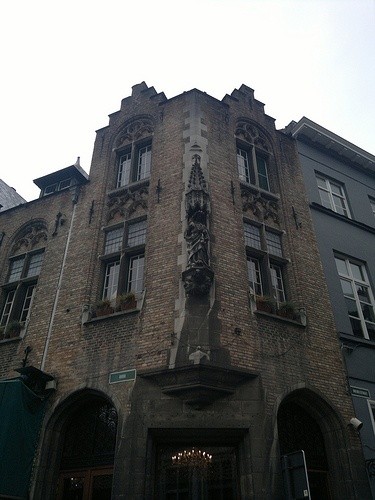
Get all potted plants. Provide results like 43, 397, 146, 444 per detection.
256, 295, 276, 313
116, 292, 138, 312
92, 299, 113, 318
5, 321, 25, 338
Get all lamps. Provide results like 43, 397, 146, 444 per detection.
347, 417, 364, 431
